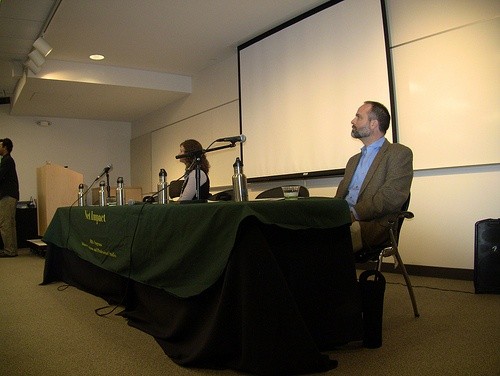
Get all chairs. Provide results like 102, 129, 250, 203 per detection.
360, 192, 421, 319
256, 184, 310, 199
211, 189, 234, 200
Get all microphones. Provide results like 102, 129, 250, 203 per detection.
97, 163, 112, 179
217, 134, 246, 142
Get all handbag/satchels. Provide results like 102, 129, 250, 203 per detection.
357, 270, 386, 348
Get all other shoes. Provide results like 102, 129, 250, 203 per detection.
0, 251, 18, 257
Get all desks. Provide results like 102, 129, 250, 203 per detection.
91, 186, 143, 204
39, 195, 362, 376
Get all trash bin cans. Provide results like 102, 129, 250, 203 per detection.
0, 200, 38, 250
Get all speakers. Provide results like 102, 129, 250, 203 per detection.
474, 218, 500, 294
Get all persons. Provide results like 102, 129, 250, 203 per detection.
0, 138, 19, 257
178, 139, 210, 200
334, 101, 414, 251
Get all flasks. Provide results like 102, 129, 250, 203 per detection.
78, 183, 87, 206
98, 181, 107, 207
157, 169, 169, 204
232, 157, 248, 202
116, 177, 126, 206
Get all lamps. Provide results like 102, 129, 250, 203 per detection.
33, 32, 53, 57
27, 49, 46, 66
23, 59, 43, 74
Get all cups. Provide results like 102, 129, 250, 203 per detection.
281, 185, 300, 201
108, 196, 116, 207
